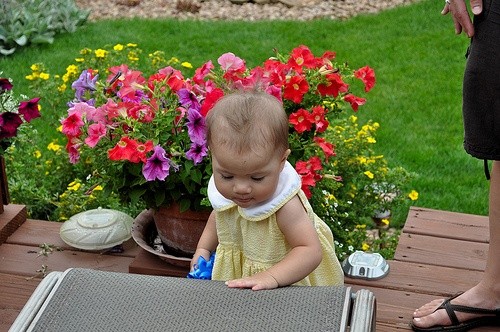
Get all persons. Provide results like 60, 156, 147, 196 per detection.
174, 90, 348, 290
402, 0, 500, 332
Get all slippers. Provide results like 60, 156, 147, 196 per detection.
409, 292, 500, 332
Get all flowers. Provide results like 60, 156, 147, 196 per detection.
3, 36, 410, 264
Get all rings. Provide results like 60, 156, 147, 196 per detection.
446, 0, 451, 6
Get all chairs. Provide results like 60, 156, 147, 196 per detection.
7, 266, 382, 332
